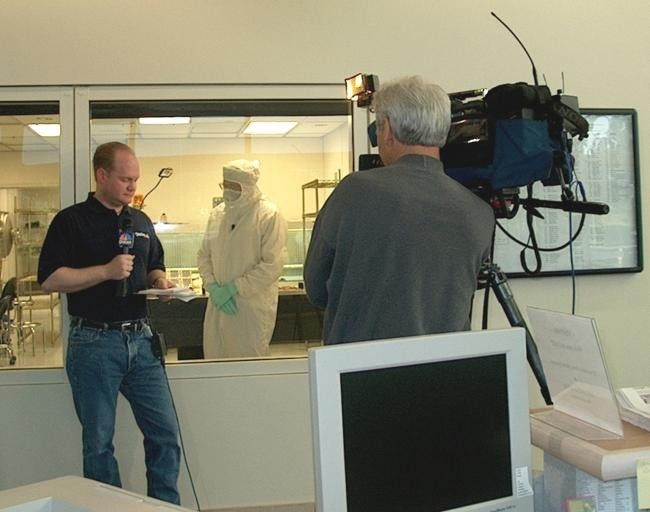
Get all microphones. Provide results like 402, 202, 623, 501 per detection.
116, 219, 134, 297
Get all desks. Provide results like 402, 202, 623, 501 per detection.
531, 405, 650, 512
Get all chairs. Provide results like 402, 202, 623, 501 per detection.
0, 277, 45, 365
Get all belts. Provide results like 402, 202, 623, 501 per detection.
72, 318, 148, 331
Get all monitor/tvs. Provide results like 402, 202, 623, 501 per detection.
307, 327, 534, 512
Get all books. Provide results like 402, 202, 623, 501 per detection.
530, 388, 650, 511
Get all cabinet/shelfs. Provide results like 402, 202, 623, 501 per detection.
302, 170, 342, 261
14, 196, 63, 347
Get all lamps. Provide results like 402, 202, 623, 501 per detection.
344, 74, 380, 107
139, 167, 173, 211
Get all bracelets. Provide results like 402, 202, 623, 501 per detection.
152, 274, 167, 289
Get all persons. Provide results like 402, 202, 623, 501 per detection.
192, 154, 289, 360
293, 75, 496, 346
33, 141, 184, 505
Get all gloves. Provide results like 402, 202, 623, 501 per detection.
206, 283, 238, 315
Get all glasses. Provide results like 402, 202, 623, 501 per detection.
219, 182, 239, 190
